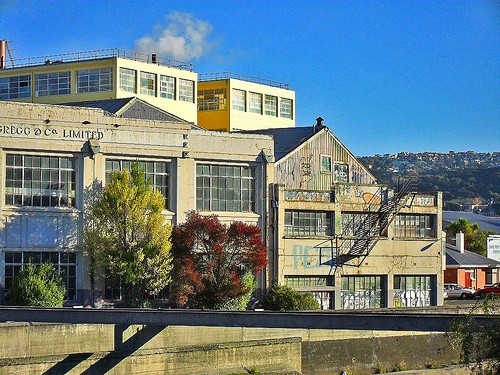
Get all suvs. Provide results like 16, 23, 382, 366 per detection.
480, 282, 500, 298
444, 284, 476, 300
